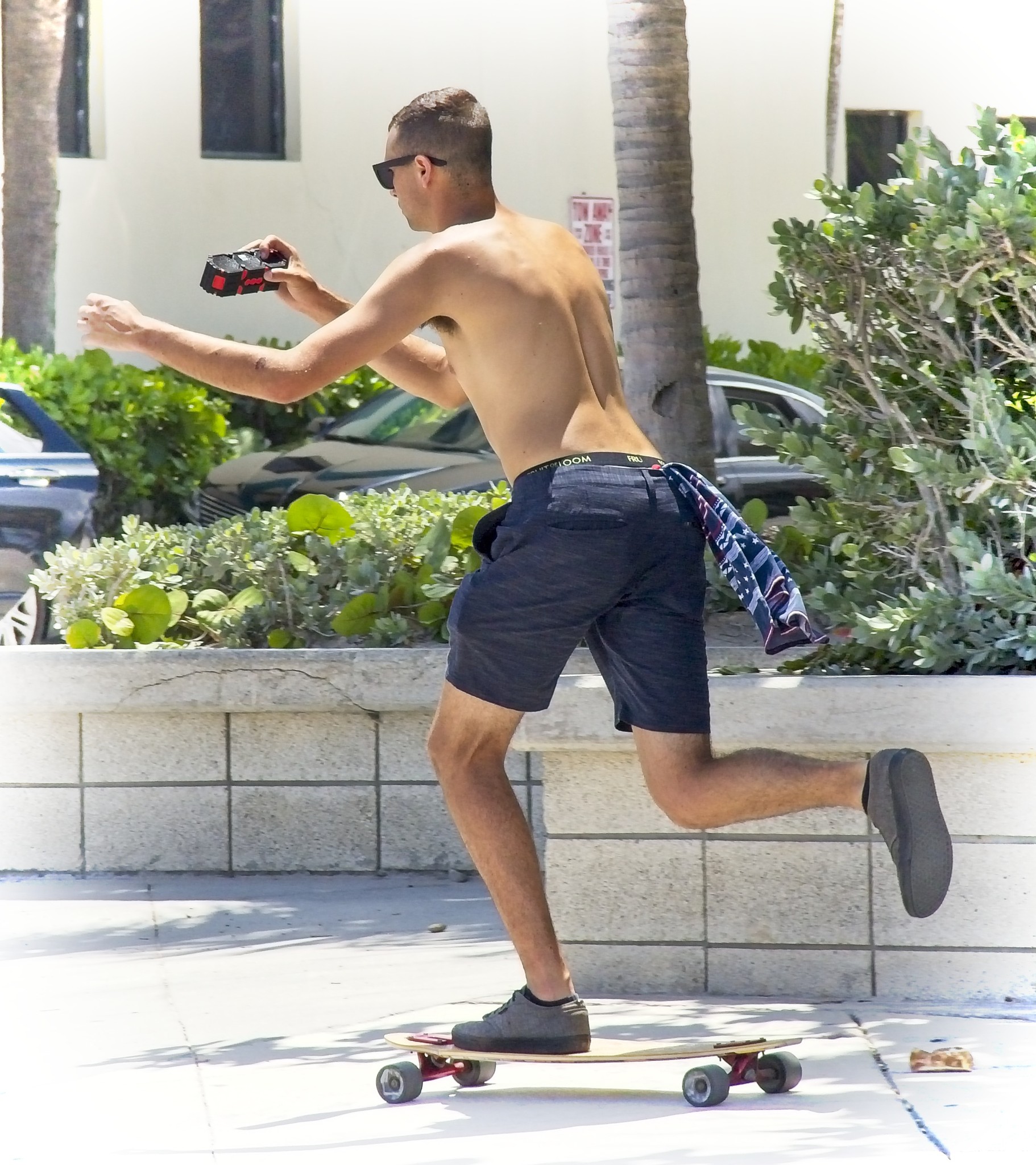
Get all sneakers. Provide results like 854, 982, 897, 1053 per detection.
864, 749, 953, 919
451, 985, 591, 1053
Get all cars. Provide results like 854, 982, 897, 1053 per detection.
192, 353, 828, 530
0, 381, 106, 648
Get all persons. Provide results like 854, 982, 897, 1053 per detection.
74, 89, 953, 1054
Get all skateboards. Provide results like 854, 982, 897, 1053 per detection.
376, 1032, 803, 1107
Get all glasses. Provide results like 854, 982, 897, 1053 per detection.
373, 155, 447, 190
